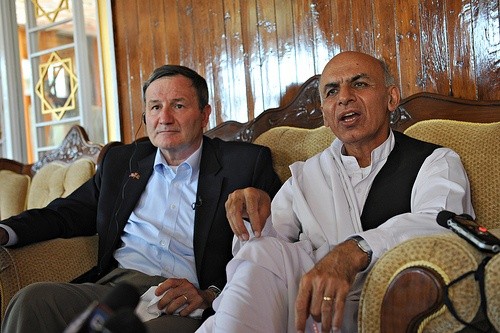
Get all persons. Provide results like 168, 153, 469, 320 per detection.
196, 50, 476, 333
0, 64, 281, 333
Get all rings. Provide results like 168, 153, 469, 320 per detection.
183, 294, 188, 303
323, 296, 331, 301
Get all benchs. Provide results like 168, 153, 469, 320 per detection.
0, 74, 500, 333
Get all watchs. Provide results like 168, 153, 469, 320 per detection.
349, 235, 372, 270
209, 288, 221, 298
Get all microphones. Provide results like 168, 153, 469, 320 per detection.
436, 210, 500, 252
78, 282, 147, 332
